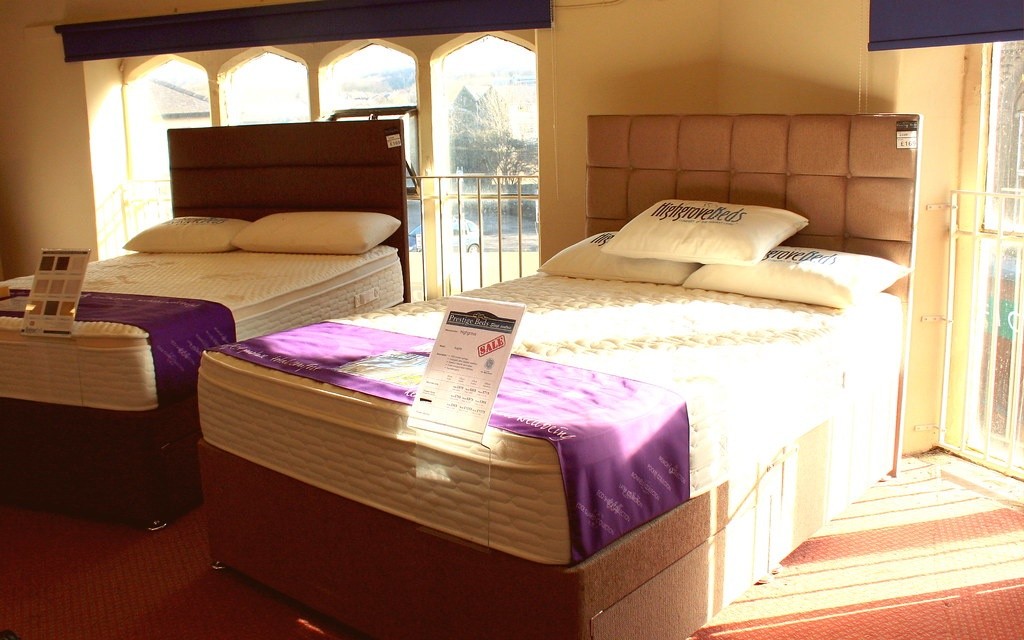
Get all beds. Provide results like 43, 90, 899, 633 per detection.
0, 121, 411, 530
197, 111, 922, 640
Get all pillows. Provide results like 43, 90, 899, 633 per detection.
123, 217, 252, 252
601, 199, 809, 266
232, 211, 401, 254
683, 246, 914, 314
537, 231, 701, 286
